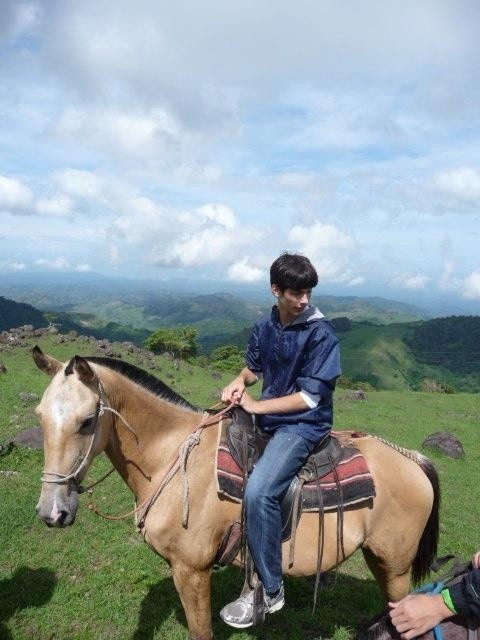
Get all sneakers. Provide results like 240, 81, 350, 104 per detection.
219, 581, 286, 629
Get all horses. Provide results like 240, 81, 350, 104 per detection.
33, 345, 442, 640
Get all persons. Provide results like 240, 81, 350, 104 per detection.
213, 251, 344, 629
383, 550, 480, 640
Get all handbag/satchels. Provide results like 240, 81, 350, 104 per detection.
354, 554, 480, 640
